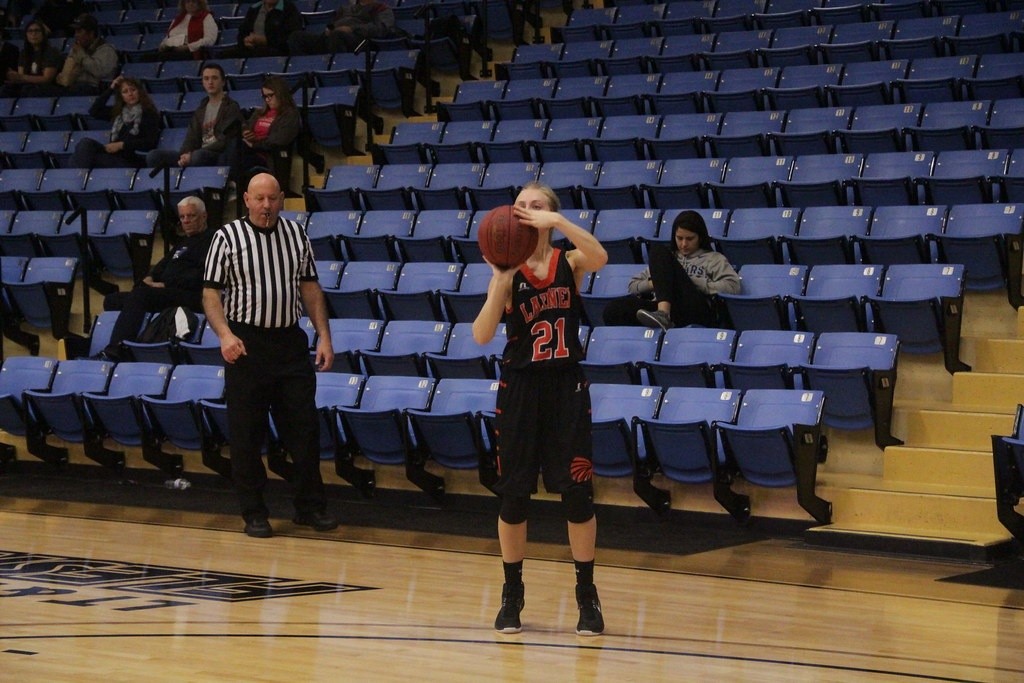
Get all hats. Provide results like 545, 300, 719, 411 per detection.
68, 13, 98, 29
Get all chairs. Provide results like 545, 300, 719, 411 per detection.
0, 0, 1024, 537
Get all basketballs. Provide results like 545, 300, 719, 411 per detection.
478, 205, 539, 266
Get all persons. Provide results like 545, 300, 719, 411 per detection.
628, 210, 741, 332
146, 63, 240, 168
103, 195, 219, 313
214, 77, 300, 202
0, 0, 119, 96
288, 0, 395, 57
201, 172, 338, 538
158, 0, 219, 61
75, 76, 166, 168
471, 181, 608, 636
237, 0, 305, 58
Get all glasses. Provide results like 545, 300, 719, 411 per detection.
262, 93, 275, 99
26, 29, 41, 34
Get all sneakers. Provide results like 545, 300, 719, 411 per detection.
293, 509, 339, 532
637, 309, 668, 333
495, 581, 525, 633
245, 519, 274, 539
574, 583, 604, 635
